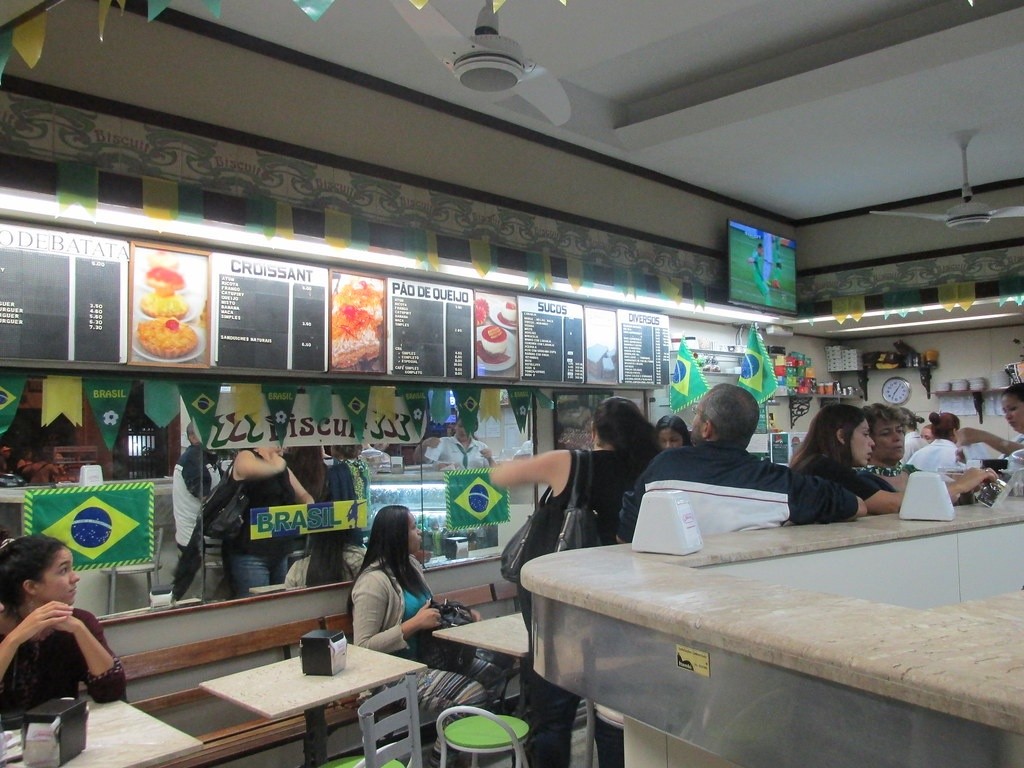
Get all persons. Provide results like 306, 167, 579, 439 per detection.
959, 383, 1024, 466
327, 445, 370, 583
414, 415, 457, 463
906, 410, 960, 504
616, 383, 867, 543
172, 420, 231, 600
921, 424, 935, 445
346, 504, 509, 768
789, 404, 999, 515
221, 447, 314, 598
489, 396, 667, 768
432, 414, 497, 470
899, 407, 928, 464
0, 530, 127, 713
853, 404, 974, 506
655, 414, 691, 450
363, 443, 390, 462
748, 231, 783, 307
18, 436, 60, 484
283, 446, 329, 586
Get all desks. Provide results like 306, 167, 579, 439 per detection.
432, 613, 530, 658
96, 597, 202, 622
248, 583, 285, 596
0, 689, 203, 768
199, 645, 428, 768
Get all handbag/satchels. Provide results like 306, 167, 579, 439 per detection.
425, 600, 477, 674
196, 449, 264, 542
501, 448, 601, 584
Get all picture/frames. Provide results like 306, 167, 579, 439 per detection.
553, 391, 614, 450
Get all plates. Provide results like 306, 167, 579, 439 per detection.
132, 258, 209, 363
476, 298, 519, 372
936, 370, 1011, 391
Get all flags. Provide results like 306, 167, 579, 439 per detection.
670, 337, 712, 414
445, 470, 510, 531
737, 326, 778, 404
23, 482, 154, 570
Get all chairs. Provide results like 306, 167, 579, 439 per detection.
357, 672, 424, 768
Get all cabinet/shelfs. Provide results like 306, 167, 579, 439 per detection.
669, 348, 745, 377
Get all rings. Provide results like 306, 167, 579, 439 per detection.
985, 468, 988, 471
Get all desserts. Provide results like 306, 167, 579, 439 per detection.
481, 301, 519, 355
138, 267, 198, 359
331, 279, 383, 367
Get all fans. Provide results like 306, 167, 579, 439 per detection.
390, 0, 572, 127
868, 132, 1024, 230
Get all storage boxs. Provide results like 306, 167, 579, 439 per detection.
775, 351, 816, 396
824, 345, 863, 372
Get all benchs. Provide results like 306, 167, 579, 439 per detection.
77, 580, 522, 768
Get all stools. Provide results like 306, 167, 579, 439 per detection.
198, 531, 225, 604
101, 527, 164, 615
437, 705, 530, 768
318, 741, 413, 768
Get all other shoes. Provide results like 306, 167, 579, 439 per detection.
430, 748, 460, 768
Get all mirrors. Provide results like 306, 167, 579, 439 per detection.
0, 374, 540, 627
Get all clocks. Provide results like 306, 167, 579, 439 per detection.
881, 376, 912, 406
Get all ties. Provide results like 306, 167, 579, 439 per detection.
457, 444, 473, 468
